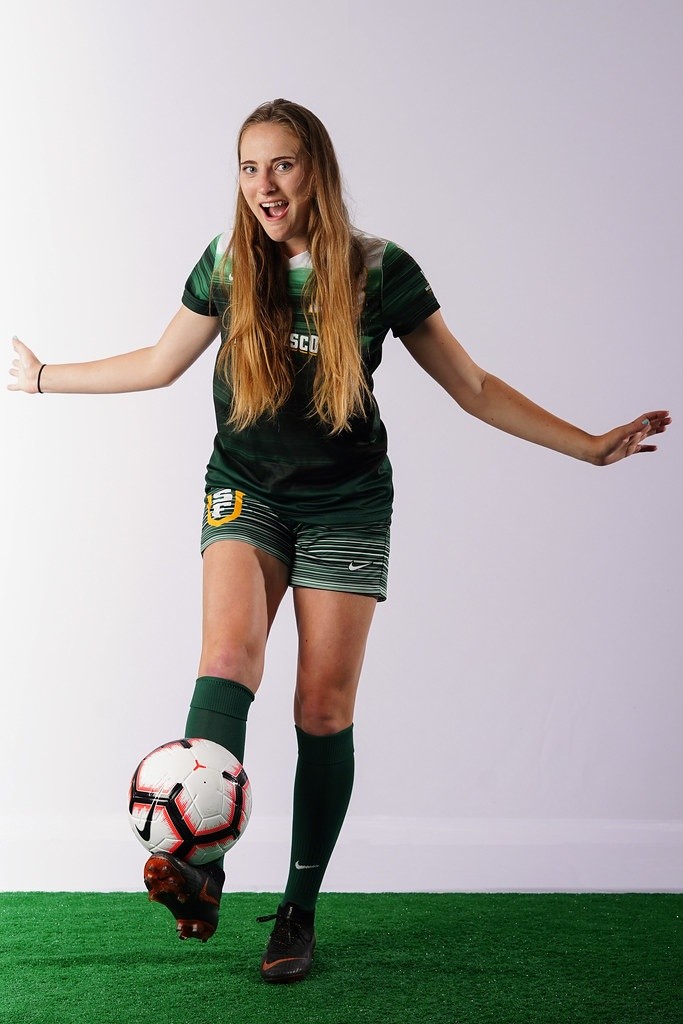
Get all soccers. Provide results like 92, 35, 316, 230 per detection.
129, 739, 253, 865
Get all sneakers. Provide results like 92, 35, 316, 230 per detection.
142, 851, 227, 940
258, 905, 318, 980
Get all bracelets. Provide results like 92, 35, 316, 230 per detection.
37, 364, 47, 394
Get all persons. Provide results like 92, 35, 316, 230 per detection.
7, 97, 672, 985
